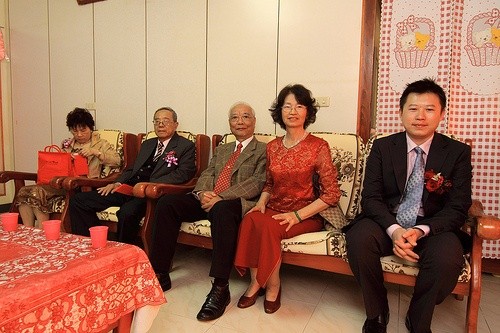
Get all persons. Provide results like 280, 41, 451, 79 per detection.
233, 86, 341, 315
147, 102, 270, 322
346, 78, 472, 333
67, 107, 196, 241
14, 108, 121, 231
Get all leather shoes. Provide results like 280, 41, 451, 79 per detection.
197, 280, 231, 320
158, 274, 171, 291
363, 305, 390, 333
405, 321, 432, 333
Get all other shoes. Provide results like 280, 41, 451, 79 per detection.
264, 278, 281, 313
237, 287, 263, 308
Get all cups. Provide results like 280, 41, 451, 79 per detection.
42, 219, 61, 240
89, 226, 109, 248
1, 213, 19, 231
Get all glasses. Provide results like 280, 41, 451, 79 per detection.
71, 128, 91, 134
230, 115, 254, 122
152, 120, 176, 126
280, 103, 308, 112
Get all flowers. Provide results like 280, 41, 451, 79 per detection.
424, 169, 452, 195
162, 149, 178, 167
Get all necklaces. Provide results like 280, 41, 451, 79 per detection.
284, 129, 308, 149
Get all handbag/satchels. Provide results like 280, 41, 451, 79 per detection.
71, 153, 89, 175
37, 145, 72, 185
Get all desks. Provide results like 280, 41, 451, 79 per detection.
0, 223, 167, 333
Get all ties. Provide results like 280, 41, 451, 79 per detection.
396, 147, 426, 229
213, 144, 243, 195
153, 141, 164, 162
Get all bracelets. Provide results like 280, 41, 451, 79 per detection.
293, 210, 302, 223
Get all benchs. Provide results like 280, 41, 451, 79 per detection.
146, 131, 500, 333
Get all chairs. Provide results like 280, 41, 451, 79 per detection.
0, 129, 211, 255
